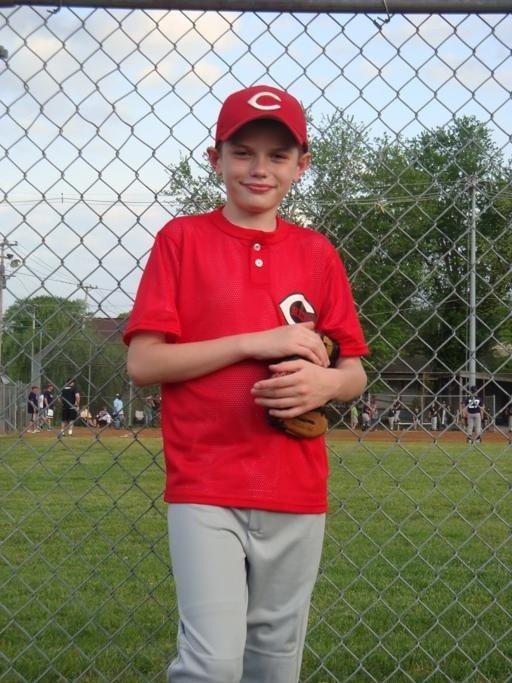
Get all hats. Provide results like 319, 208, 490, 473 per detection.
215, 85, 308, 156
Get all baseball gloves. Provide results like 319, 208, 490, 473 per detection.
267, 334, 340, 439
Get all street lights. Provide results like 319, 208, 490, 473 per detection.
0, 241, 20, 313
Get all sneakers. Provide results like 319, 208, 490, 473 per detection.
62, 434, 72, 437
27, 427, 53, 432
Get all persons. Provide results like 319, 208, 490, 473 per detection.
347, 382, 491, 444
24, 376, 160, 439
118, 85, 372, 683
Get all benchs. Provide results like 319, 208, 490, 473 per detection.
394, 422, 433, 432
